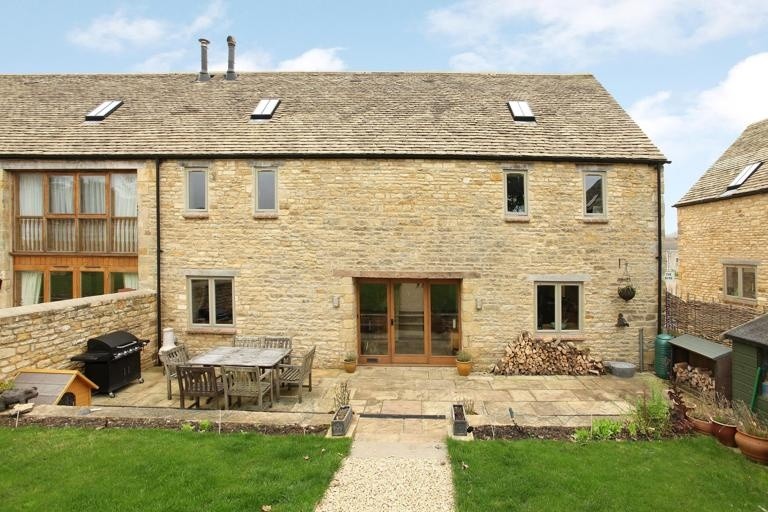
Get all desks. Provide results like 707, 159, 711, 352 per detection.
184, 346, 293, 406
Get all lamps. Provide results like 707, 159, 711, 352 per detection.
331, 295, 340, 309
474, 297, 483, 311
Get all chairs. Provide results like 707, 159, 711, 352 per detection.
260, 338, 292, 388
220, 364, 273, 412
175, 365, 223, 411
231, 335, 262, 350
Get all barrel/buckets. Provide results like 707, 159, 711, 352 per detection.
653, 333, 674, 380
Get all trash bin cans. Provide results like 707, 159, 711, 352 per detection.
655, 334, 673, 380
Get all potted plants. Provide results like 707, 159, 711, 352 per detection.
616, 258, 636, 302
330, 379, 353, 436
708, 395, 736, 447
730, 399, 767, 464
451, 404, 467, 435
684, 399, 712, 437
342, 350, 357, 374
454, 349, 472, 377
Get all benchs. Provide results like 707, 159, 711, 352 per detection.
160, 344, 193, 402
275, 345, 316, 404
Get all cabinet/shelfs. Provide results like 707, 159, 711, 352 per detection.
83, 351, 145, 399
